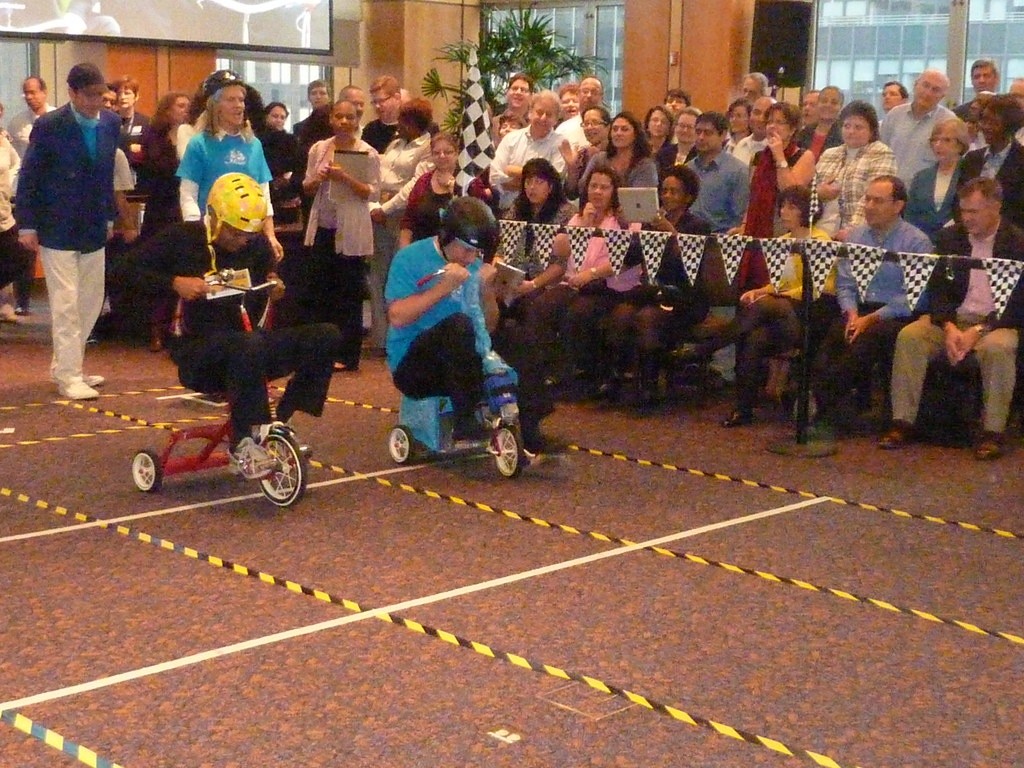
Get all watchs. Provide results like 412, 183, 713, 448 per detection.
775, 160, 789, 171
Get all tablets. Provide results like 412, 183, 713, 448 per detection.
618, 187, 659, 223
492, 261, 525, 307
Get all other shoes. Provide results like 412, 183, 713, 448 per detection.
57, 378, 99, 401
879, 421, 914, 450
518, 354, 862, 428
83, 375, 104, 387
150, 323, 162, 352
976, 429, 1005, 461
521, 428, 549, 460
451, 421, 490, 449
15, 303, 32, 315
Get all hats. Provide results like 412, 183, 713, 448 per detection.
66, 63, 109, 97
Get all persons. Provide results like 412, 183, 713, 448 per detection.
2, 55, 1024, 479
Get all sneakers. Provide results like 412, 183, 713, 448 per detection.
226, 435, 274, 479
276, 422, 313, 458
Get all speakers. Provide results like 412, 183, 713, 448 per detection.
750, 0, 816, 88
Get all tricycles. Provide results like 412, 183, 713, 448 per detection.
388, 265, 533, 478
132, 279, 312, 509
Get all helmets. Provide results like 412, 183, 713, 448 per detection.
207, 172, 266, 232
202, 69, 246, 101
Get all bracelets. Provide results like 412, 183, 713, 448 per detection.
445, 179, 455, 188
591, 267, 598, 281
531, 280, 539, 292
975, 323, 986, 336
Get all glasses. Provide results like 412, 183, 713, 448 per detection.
694, 128, 719, 136
371, 93, 393, 105
929, 135, 960, 145
580, 119, 608, 128
677, 122, 696, 131
